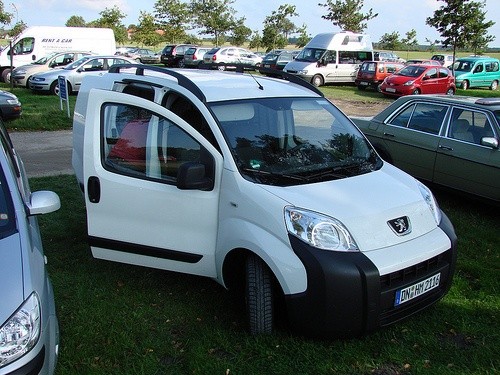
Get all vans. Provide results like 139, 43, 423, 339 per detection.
283, 31, 375, 88
0, 26, 116, 84
355, 61, 408, 90
448, 56, 500, 91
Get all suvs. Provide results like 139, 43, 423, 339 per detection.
183, 47, 217, 68
159, 44, 199, 67
202, 47, 263, 72
259, 53, 306, 78
75, 64, 458, 339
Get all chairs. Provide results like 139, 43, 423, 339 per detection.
92, 59, 102, 70
450, 118, 477, 143
62, 54, 72, 65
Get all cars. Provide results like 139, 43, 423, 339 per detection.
381, 65, 456, 98
0, 90, 64, 375
10, 51, 105, 88
28, 55, 143, 97
332, 95, 500, 202
374, 51, 407, 65
406, 59, 443, 69
124, 48, 160, 64
431, 55, 468, 69
254, 50, 302, 60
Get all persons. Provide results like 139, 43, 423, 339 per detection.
107, 106, 150, 169
